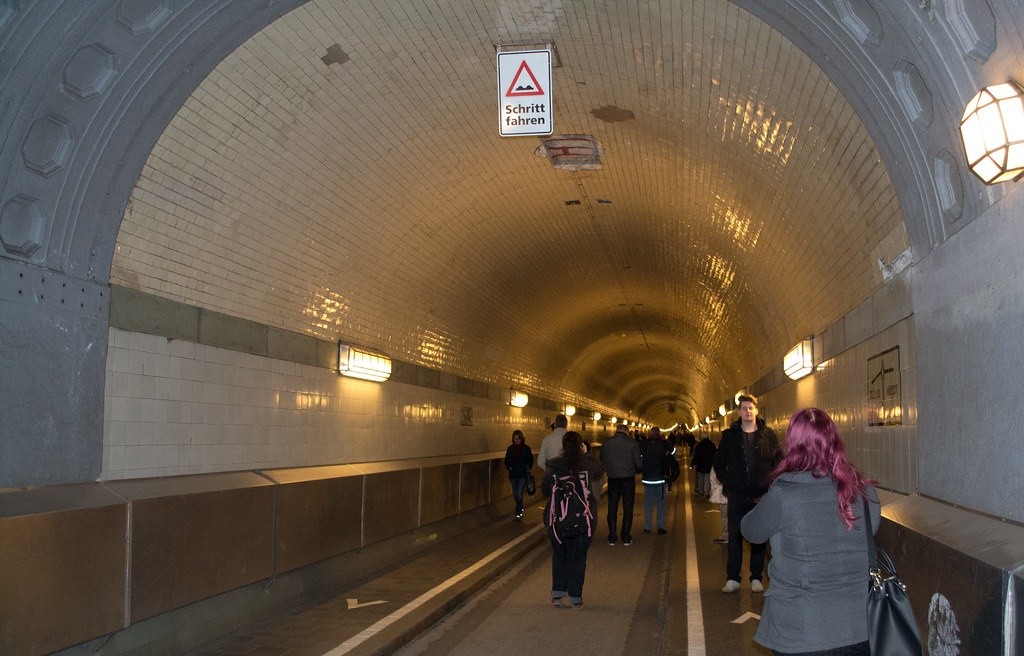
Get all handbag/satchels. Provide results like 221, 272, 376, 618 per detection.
526, 469, 536, 496
868, 568, 923, 656
662, 451, 681, 483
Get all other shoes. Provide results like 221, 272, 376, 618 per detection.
608, 541, 615, 545
624, 540, 633, 546
572, 602, 583, 609
752, 579, 764, 593
713, 538, 729, 543
552, 597, 562, 605
705, 495, 710, 501
722, 579, 740, 593
514, 512, 522, 521
644, 529, 651, 533
698, 493, 703, 497
658, 528, 666, 534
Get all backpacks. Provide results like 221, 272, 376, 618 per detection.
548, 475, 593, 544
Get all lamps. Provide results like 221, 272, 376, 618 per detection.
560, 401, 730, 432
784, 335, 814, 380
510, 390, 529, 408
735, 385, 750, 406
958, 78, 1024, 186
336, 342, 391, 382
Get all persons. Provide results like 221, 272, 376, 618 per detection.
627, 428, 647, 443
667, 427, 696, 455
540, 430, 603, 610
504, 430, 534, 523
740, 409, 880, 656
713, 400, 785, 597
690, 431, 719, 502
639, 426, 682, 536
598, 423, 643, 547
707, 466, 729, 545
536, 413, 569, 472
